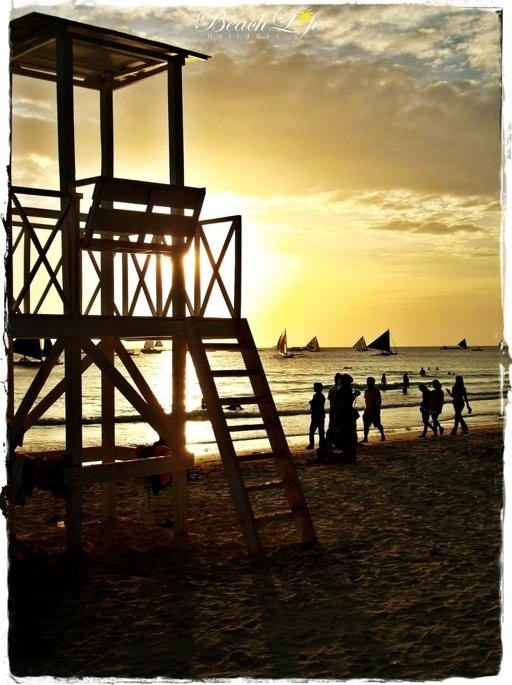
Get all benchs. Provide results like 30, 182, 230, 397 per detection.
78, 177, 205, 258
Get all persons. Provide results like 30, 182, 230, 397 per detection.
306, 366, 473, 451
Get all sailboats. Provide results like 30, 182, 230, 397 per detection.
140, 338, 166, 353
352, 328, 399, 356
13, 338, 63, 365
440, 339, 470, 352
276, 328, 320, 358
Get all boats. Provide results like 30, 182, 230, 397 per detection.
114, 349, 140, 356
